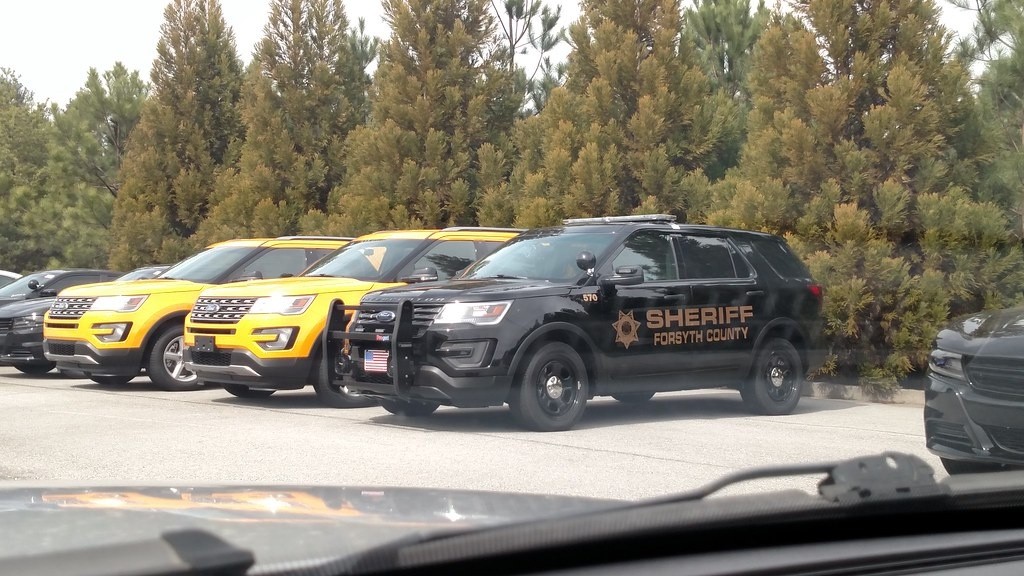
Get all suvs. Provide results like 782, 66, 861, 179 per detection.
178, 225, 532, 408
923, 303, 1024, 477
1, 267, 130, 377
328, 214, 827, 433
42, 235, 389, 393
